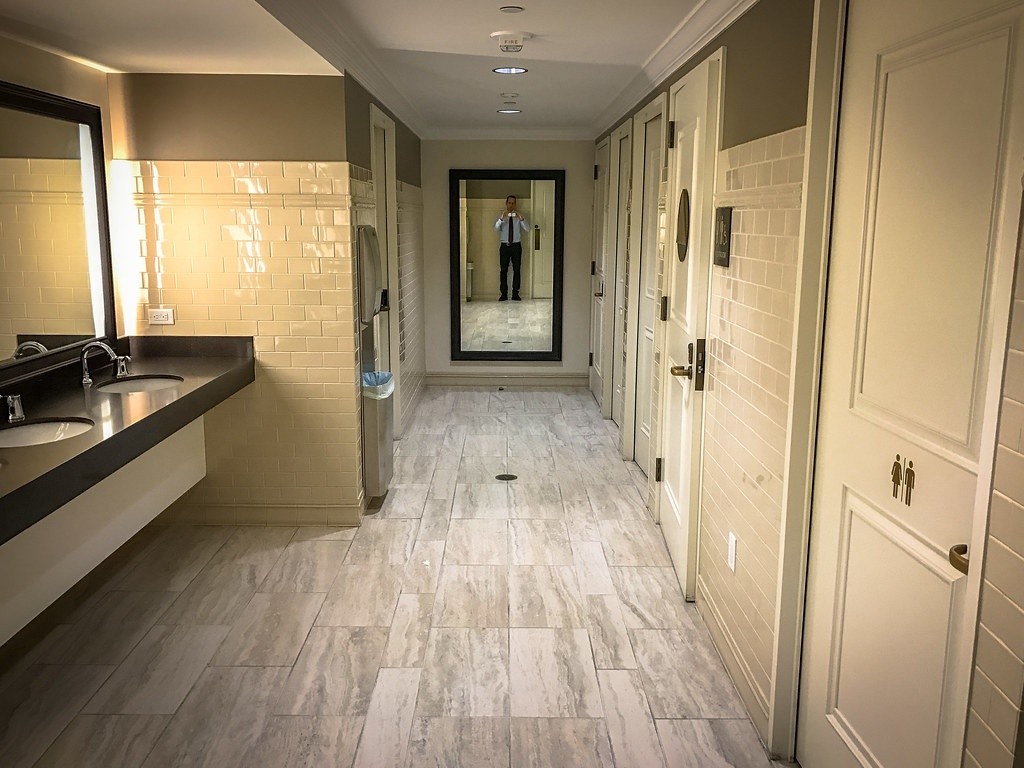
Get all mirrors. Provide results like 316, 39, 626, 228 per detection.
0, 80, 120, 388
448, 168, 566, 362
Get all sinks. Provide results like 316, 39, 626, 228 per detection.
93, 374, 184, 393
0, 416, 96, 448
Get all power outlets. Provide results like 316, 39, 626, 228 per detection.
726, 532, 738, 573
148, 308, 175, 325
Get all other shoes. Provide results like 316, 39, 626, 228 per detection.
499, 295, 509, 301
512, 295, 521, 301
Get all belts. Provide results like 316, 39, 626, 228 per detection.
501, 242, 521, 246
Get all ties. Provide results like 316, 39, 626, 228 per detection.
508, 217, 514, 243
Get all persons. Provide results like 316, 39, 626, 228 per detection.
495, 196, 530, 301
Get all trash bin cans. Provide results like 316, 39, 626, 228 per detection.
466, 263, 472, 303
363, 372, 394, 498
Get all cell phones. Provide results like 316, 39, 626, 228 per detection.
508, 213, 516, 217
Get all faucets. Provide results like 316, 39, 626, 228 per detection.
80, 341, 117, 384
14, 340, 48, 356
